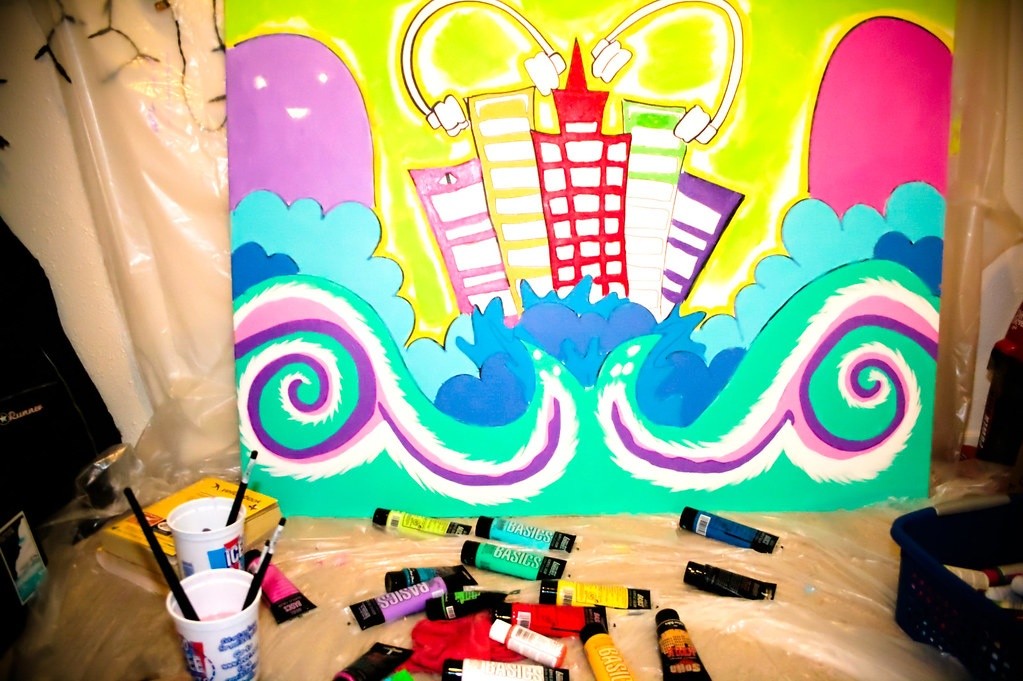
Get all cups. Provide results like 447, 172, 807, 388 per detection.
166, 496, 246, 576
165, 568, 262, 681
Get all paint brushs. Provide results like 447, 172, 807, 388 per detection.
226, 450, 259, 529
242, 518, 286, 611
253, 539, 271, 577
123, 486, 201, 622
